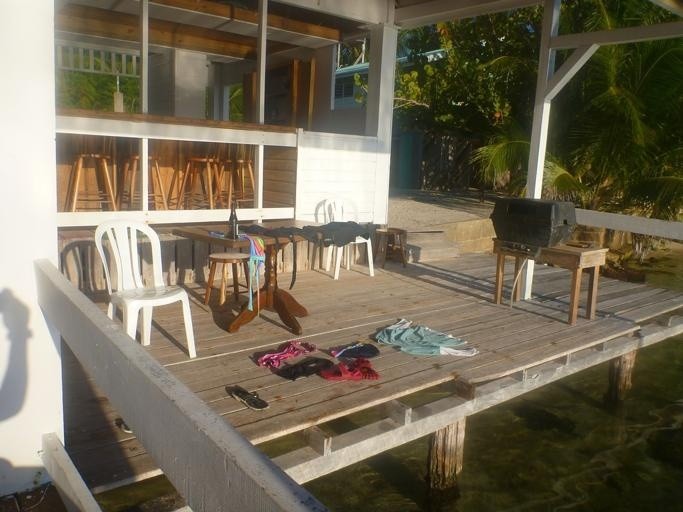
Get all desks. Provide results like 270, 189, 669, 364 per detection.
173, 221, 368, 334
490, 238, 609, 327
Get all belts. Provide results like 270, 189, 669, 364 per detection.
288, 233, 298, 290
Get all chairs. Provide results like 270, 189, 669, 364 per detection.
215, 144, 254, 208
93, 218, 198, 360
325, 197, 374, 280
176, 141, 224, 210
69, 133, 118, 212
118, 138, 169, 211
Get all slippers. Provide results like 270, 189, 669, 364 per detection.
230, 386, 270, 412
320, 364, 381, 382
283, 358, 332, 380
338, 340, 381, 357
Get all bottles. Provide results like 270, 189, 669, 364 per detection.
228, 203, 238, 239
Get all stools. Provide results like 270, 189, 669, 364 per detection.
205, 253, 250, 309
374, 227, 407, 270
